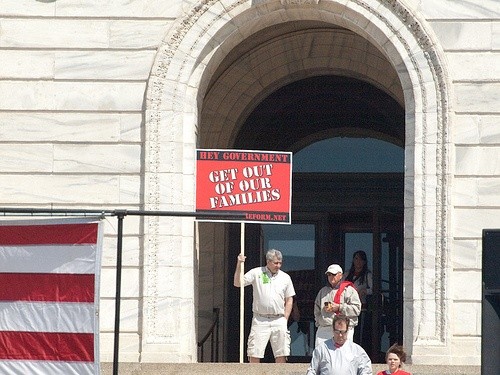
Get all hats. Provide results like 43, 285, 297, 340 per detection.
325, 264, 343, 274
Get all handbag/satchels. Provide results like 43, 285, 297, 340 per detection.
367, 295, 374, 309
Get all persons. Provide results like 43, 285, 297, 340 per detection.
342, 251, 374, 363
233, 248, 296, 363
307, 312, 374, 375
313, 264, 362, 349
376, 342, 411, 375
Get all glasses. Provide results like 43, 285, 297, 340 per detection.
334, 329, 347, 334
328, 273, 338, 276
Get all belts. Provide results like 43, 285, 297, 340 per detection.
253, 313, 284, 318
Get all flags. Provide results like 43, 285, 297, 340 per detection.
0, 216, 108, 375
